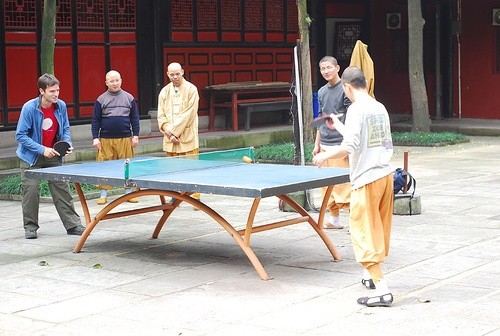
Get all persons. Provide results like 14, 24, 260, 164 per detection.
311, 56, 352, 229
15, 73, 86, 239
91, 70, 140, 204
312, 66, 393, 306
157, 62, 201, 210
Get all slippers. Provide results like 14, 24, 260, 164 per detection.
323, 220, 343, 229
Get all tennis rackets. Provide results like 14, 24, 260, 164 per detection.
53, 141, 70, 157
309, 113, 343, 128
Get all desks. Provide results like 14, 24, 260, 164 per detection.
223, 96, 292, 131
204, 80, 291, 132
25, 155, 351, 281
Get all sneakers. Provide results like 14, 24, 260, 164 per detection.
128, 196, 138, 203
97, 197, 107, 204
67, 225, 86, 235
25, 229, 37, 239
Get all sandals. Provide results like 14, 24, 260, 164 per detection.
357, 293, 393, 307
362, 279, 376, 289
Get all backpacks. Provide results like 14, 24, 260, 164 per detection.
394, 168, 415, 199
